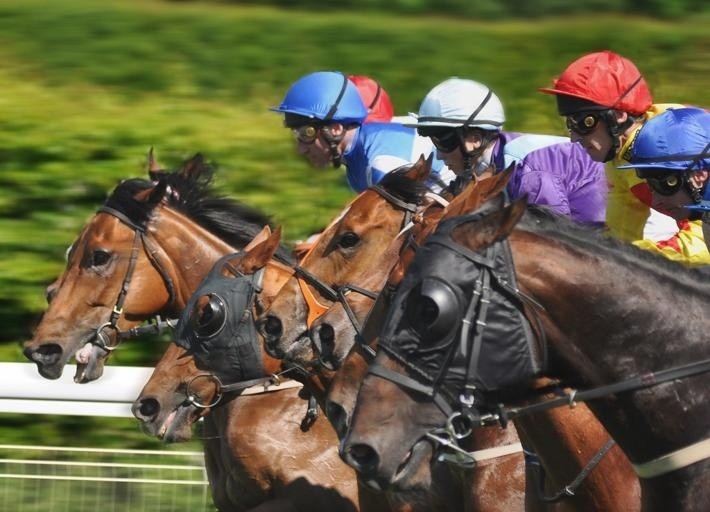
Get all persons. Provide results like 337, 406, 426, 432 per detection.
265, 71, 456, 197
536, 49, 709, 265
612, 108, 710, 255
397, 75, 610, 229
345, 74, 419, 125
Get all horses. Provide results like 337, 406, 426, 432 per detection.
338, 192, 710, 512
20, 146, 644, 512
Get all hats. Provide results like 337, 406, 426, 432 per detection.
268, 71, 394, 127
536, 50, 652, 115
616, 108, 710, 174
401, 76, 507, 133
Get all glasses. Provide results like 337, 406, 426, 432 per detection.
291, 124, 323, 145
646, 172, 687, 197
566, 112, 608, 136
430, 134, 466, 154
